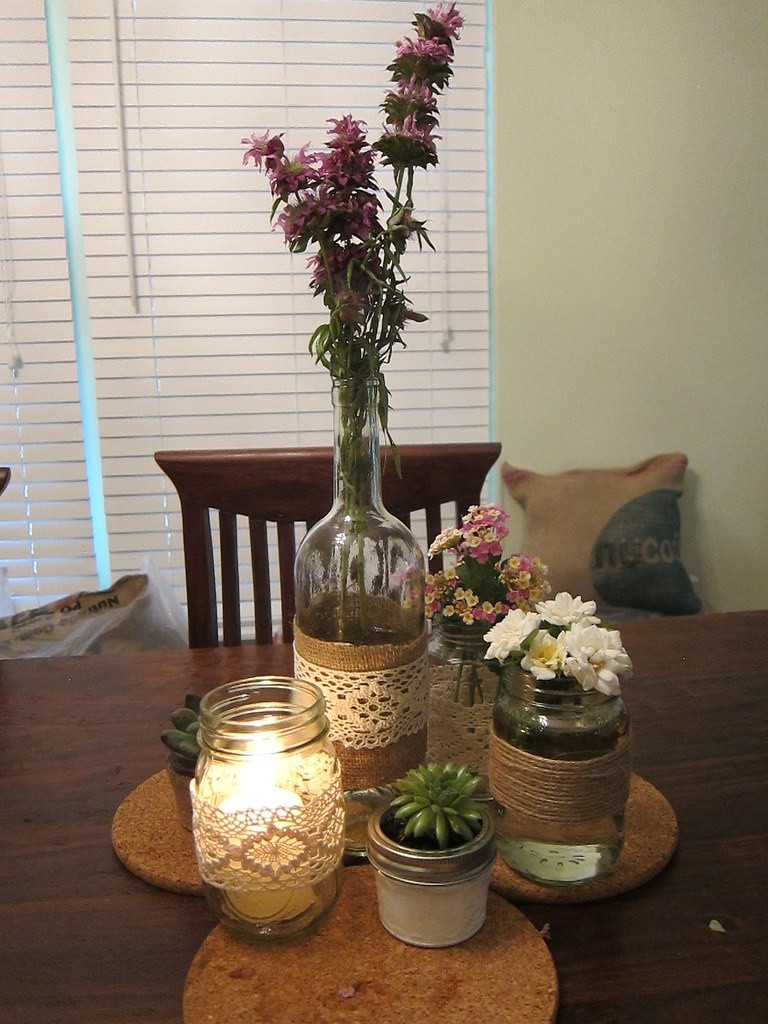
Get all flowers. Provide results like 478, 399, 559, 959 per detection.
482, 590, 634, 696
427, 505, 553, 626
238, 2, 467, 376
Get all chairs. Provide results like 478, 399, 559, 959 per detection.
154, 440, 502, 647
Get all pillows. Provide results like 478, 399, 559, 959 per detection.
497, 452, 703, 621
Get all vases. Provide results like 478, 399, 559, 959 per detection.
291, 372, 429, 862
187, 675, 347, 946
425, 623, 502, 802
487, 663, 634, 887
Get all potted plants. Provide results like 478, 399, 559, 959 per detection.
110, 691, 216, 898
364, 759, 498, 949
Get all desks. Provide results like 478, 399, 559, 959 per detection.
0, 609, 767, 1022
